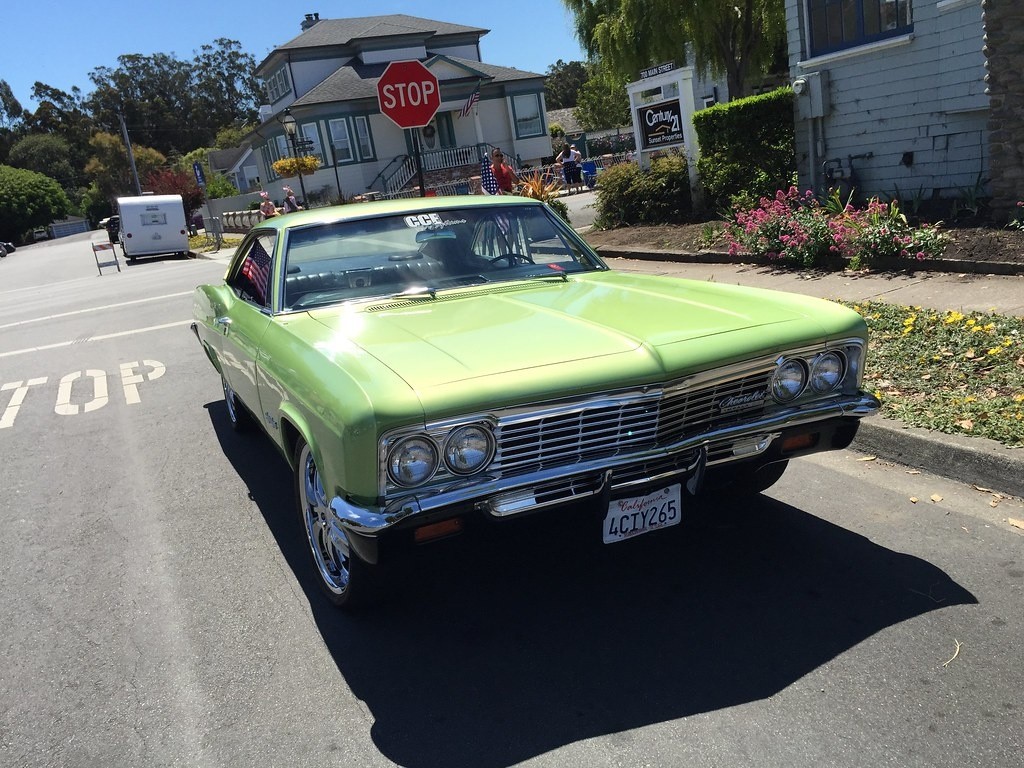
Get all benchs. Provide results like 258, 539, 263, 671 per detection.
284, 263, 446, 303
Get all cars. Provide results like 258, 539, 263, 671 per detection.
32, 229, 49, 243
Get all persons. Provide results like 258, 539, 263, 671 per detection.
556, 143, 584, 195
260, 197, 277, 220
283, 189, 302, 214
489, 147, 520, 196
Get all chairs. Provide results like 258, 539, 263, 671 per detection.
582, 161, 603, 188
553, 163, 575, 192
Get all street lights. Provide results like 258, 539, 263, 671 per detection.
282, 108, 309, 210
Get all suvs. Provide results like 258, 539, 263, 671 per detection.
106, 215, 120, 244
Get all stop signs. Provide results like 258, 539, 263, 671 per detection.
377, 59, 442, 130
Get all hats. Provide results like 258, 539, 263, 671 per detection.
571, 144, 576, 148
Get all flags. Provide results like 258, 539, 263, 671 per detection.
480, 152, 511, 236
457, 84, 480, 120
242, 239, 271, 297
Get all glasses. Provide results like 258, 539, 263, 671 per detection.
495, 154, 503, 158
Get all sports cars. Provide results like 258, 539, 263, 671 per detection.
190, 195, 882, 614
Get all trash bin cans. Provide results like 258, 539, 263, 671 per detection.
582, 161, 597, 189
